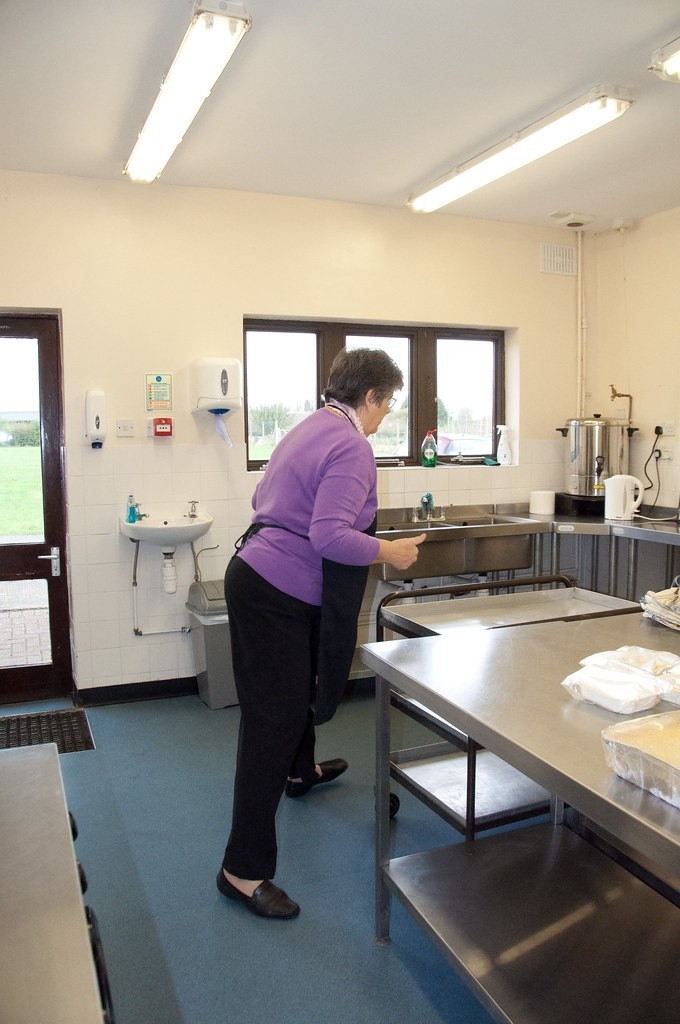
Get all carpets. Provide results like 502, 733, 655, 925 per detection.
0, 708, 96, 755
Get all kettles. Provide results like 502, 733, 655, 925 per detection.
603, 474, 644, 520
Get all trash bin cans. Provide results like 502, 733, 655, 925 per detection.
185, 579, 239, 709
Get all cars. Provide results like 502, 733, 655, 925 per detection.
391, 433, 492, 454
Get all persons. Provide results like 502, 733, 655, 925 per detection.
217, 348, 426, 919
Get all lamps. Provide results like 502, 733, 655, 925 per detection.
122, 0, 252, 185
407, 84, 635, 213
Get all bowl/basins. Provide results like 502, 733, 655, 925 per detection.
574, 646, 680, 713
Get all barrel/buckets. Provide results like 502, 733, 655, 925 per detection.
555, 414, 640, 497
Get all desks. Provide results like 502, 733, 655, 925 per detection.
0, 743, 105, 1024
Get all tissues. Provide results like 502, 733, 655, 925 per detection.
188, 357, 242, 449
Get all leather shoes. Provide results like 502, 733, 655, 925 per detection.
285, 757, 348, 798
216, 867, 300, 919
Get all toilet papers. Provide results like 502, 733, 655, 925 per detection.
529, 491, 555, 514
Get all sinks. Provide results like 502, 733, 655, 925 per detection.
369, 522, 465, 582
439, 517, 532, 575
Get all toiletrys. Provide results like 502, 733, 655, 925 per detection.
127, 495, 142, 523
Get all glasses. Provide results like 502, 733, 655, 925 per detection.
376, 397, 397, 408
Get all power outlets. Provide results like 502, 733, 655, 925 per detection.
658, 424, 675, 436
657, 449, 672, 460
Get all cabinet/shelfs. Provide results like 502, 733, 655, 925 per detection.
349, 536, 680, 1024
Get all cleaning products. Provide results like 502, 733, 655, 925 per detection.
421, 429, 437, 467
86, 391, 107, 449
496, 424, 511, 465
119, 513, 213, 547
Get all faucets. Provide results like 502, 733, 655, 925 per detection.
136, 503, 149, 519
188, 501, 199, 518
596, 455, 605, 476
610, 383, 618, 401
420, 492, 434, 520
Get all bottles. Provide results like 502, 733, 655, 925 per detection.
126, 495, 136, 523
421, 429, 437, 467
